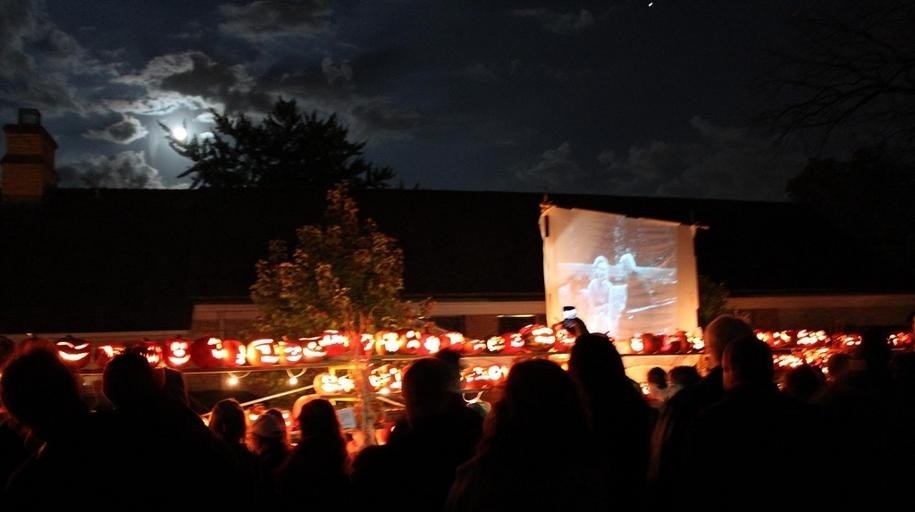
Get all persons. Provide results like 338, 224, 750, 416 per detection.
615, 252, 654, 337
583, 254, 615, 321
0, 315, 915, 512
